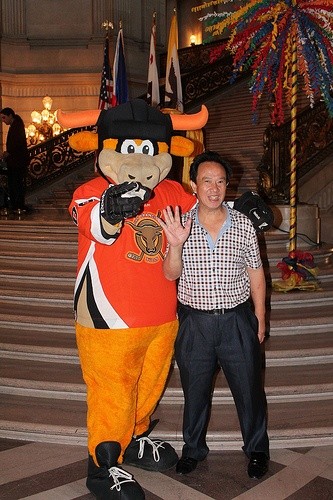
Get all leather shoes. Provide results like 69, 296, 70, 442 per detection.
247, 458, 268, 480
175, 454, 208, 475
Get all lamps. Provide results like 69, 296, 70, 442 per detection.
190, 34, 196, 47
24, 95, 72, 147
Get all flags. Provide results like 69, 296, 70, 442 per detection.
164, 7, 184, 111
113, 20, 130, 106
147, 10, 161, 109
97, 18, 113, 110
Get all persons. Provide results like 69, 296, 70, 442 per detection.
156, 152, 271, 480
0, 107, 29, 212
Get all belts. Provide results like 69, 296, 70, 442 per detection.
179, 300, 251, 315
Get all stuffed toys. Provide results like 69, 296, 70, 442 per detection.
69, 98, 274, 500
